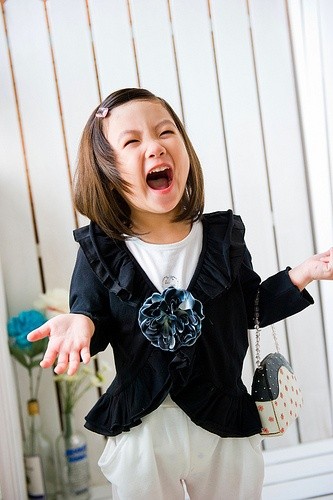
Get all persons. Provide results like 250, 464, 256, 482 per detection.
27, 88, 333, 499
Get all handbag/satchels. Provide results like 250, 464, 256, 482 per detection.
251, 284, 305, 437
